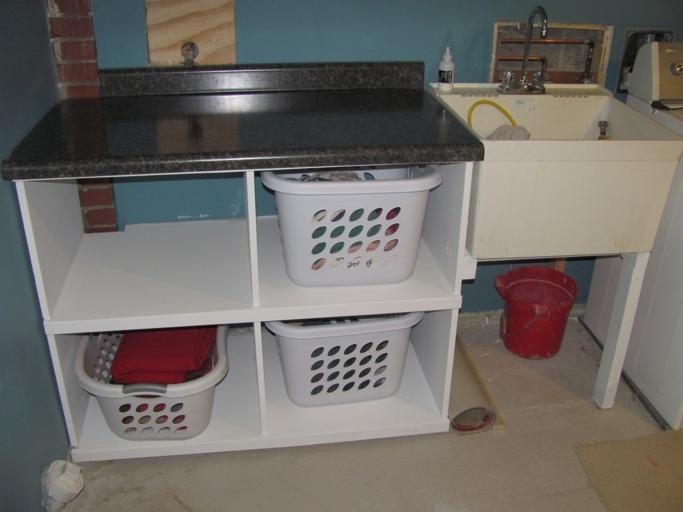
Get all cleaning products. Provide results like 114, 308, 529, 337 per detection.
437, 46, 455, 92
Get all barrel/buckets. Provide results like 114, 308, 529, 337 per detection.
493, 267, 579, 361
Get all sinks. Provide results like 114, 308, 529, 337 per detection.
428, 81, 683, 261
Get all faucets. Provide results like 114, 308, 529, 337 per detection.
520, 6, 548, 82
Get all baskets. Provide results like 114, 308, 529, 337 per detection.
261, 164, 442, 287
264, 312, 423, 407
74, 326, 228, 440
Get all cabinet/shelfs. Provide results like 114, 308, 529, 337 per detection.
0, 162, 485, 462
